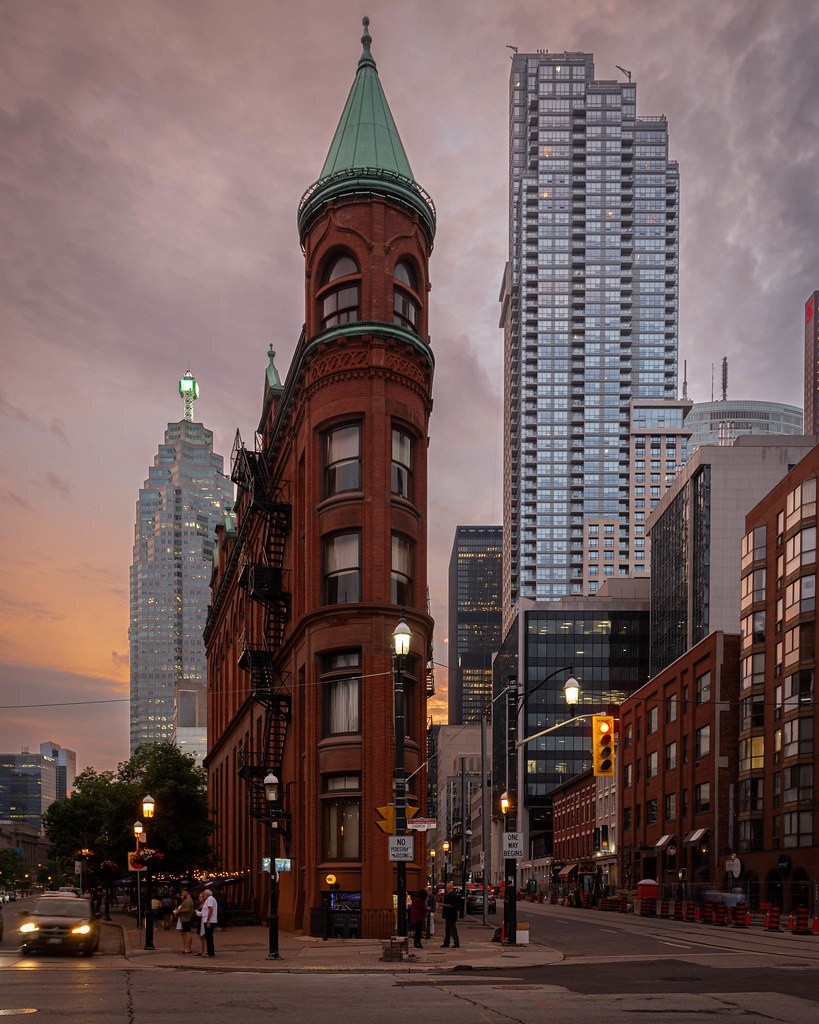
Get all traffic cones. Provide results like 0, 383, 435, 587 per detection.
762, 910, 770, 927
785, 912, 795, 930
745, 912, 752, 927
811, 913, 819, 933
684, 905, 733, 924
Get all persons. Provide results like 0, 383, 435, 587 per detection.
83, 890, 91, 899
406, 883, 460, 949
677, 883, 685, 901
152, 888, 218, 958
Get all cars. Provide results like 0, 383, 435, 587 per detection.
453, 886, 496, 915
0, 891, 16, 903
21, 887, 103, 956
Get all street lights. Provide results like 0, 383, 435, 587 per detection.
391, 603, 414, 937
443, 840, 449, 894
134, 819, 144, 928
264, 769, 283, 960
430, 849, 435, 895
143, 791, 156, 952
502, 663, 582, 947
501, 792, 509, 878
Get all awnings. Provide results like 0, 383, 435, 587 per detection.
684, 827, 710, 848
655, 834, 677, 852
560, 864, 577, 878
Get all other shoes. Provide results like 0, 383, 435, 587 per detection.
203, 953, 216, 958
196, 952, 203, 956
414, 943, 424, 949
452, 944, 461, 949
440, 943, 450, 948
179, 949, 192, 954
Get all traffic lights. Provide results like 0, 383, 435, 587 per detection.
374, 806, 396, 834
591, 716, 615, 776
405, 805, 422, 835
